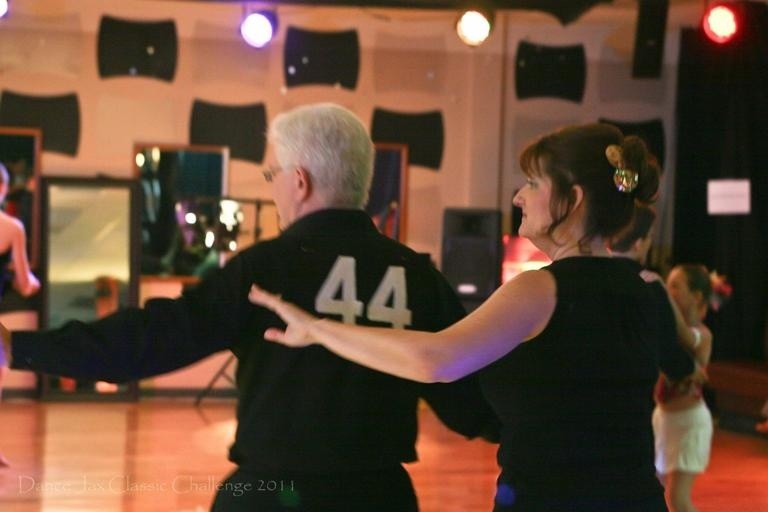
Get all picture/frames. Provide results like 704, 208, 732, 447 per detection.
366, 143, 408, 245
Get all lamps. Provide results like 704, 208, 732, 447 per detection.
241, 9, 277, 48
456, 6, 495, 47
702, 1, 752, 44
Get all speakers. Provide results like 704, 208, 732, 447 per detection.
441, 208, 504, 302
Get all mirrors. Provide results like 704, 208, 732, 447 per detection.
39, 175, 141, 402
0, 126, 43, 399
133, 143, 230, 284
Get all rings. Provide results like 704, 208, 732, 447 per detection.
277, 292, 282, 299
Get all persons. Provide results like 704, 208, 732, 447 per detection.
0, 161, 41, 470
608, 200, 710, 386
639, 262, 732, 512
248, 124, 669, 512
0, 102, 504, 512
